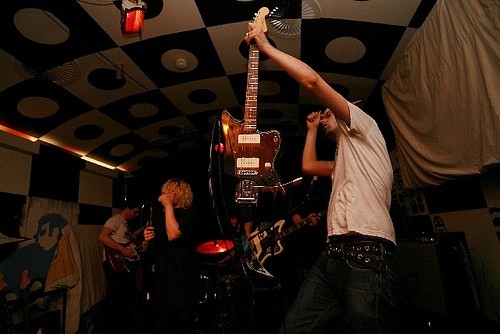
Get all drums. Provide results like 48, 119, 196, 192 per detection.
220, 275, 240, 296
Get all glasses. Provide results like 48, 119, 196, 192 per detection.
132, 209, 139, 214
325, 111, 332, 118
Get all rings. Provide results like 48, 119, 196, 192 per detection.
246, 33, 249, 37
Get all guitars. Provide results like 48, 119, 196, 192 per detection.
242, 207, 327, 278
107, 242, 141, 276
220, 7, 291, 222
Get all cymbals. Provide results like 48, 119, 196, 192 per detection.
195, 239, 235, 255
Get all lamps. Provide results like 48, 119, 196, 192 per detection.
121, 0, 148, 34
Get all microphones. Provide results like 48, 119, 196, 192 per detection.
140, 198, 158, 206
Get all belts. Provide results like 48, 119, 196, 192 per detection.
327, 240, 392, 273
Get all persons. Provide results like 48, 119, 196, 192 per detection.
97, 200, 149, 307
143, 178, 204, 334
244, 21, 399, 334
243, 191, 321, 239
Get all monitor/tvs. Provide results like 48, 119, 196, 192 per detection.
14, 309, 61, 334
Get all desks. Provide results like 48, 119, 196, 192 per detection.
396, 235, 474, 317
